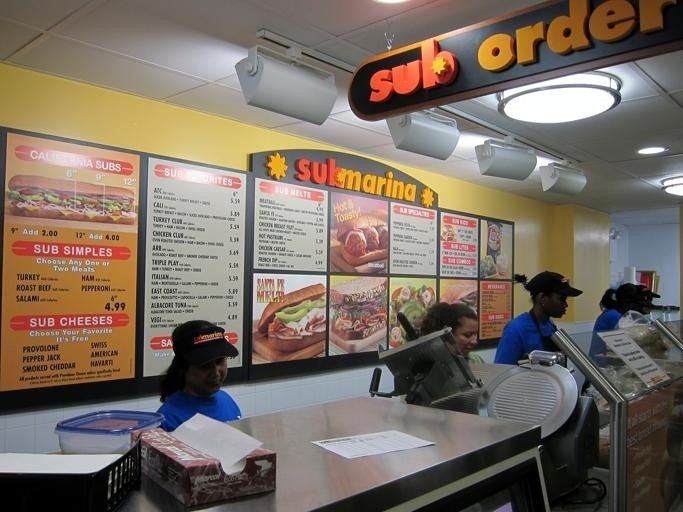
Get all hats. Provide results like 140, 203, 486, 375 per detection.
173, 326, 239, 367
528, 272, 584, 297
641, 287, 661, 300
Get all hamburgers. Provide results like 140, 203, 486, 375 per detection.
257, 283, 327, 352
329, 278, 388, 353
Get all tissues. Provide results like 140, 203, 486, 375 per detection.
130, 412, 277, 507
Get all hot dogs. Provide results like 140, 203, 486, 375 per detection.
337, 209, 388, 265
7, 174, 137, 227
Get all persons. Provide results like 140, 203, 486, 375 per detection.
581, 282, 647, 394
400, 300, 490, 409
494, 269, 584, 366
154, 320, 243, 432
641, 287, 660, 315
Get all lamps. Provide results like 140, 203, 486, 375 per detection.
494, 65, 622, 123
660, 175, 682, 198
236, 28, 588, 197
